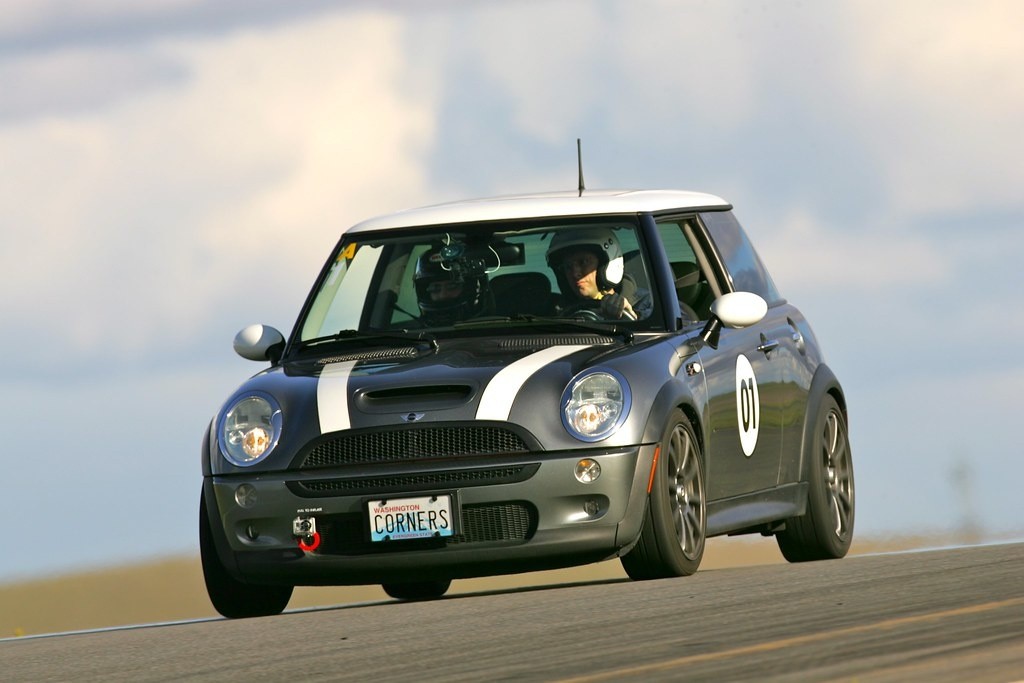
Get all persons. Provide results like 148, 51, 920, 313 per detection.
412, 243, 495, 318
546, 229, 686, 322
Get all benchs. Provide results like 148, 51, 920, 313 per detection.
487, 261, 715, 314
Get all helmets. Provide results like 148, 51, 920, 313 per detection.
412, 244, 489, 325
545, 228, 625, 296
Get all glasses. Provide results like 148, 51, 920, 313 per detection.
426, 280, 465, 293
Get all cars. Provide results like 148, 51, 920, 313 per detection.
198, 135, 857, 619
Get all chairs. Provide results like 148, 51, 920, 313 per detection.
622, 250, 654, 310
